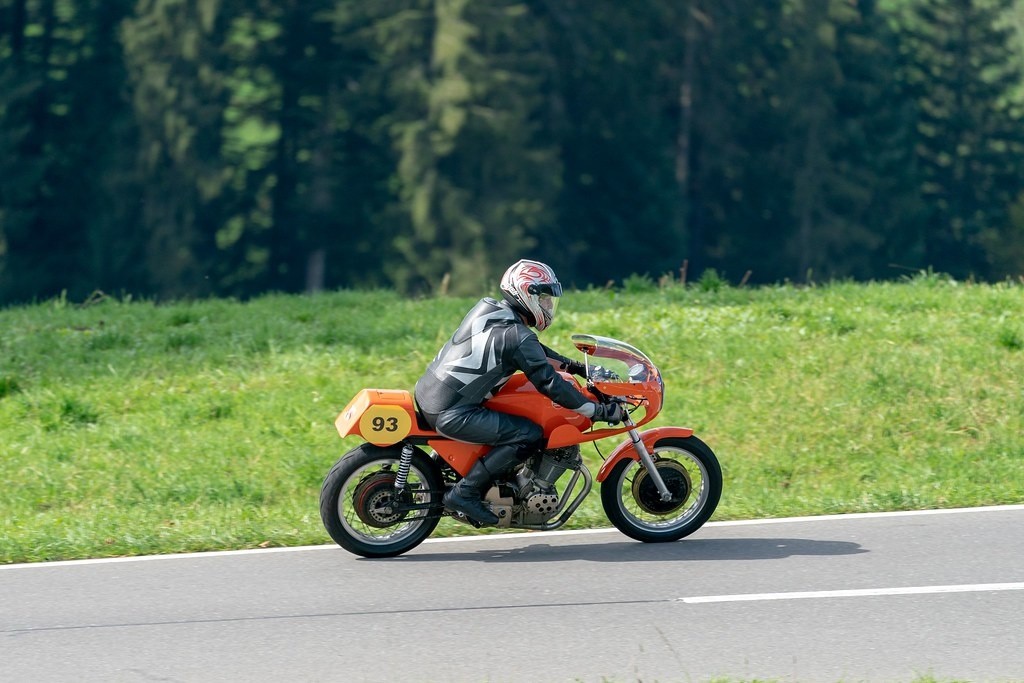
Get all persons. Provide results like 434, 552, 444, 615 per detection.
412, 258, 625, 525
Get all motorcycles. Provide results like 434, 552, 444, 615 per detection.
320, 333, 722, 558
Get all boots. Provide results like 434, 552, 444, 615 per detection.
442, 456, 501, 525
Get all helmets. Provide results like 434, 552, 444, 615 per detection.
499, 259, 563, 332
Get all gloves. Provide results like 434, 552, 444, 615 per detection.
592, 403, 624, 424
568, 359, 597, 379
